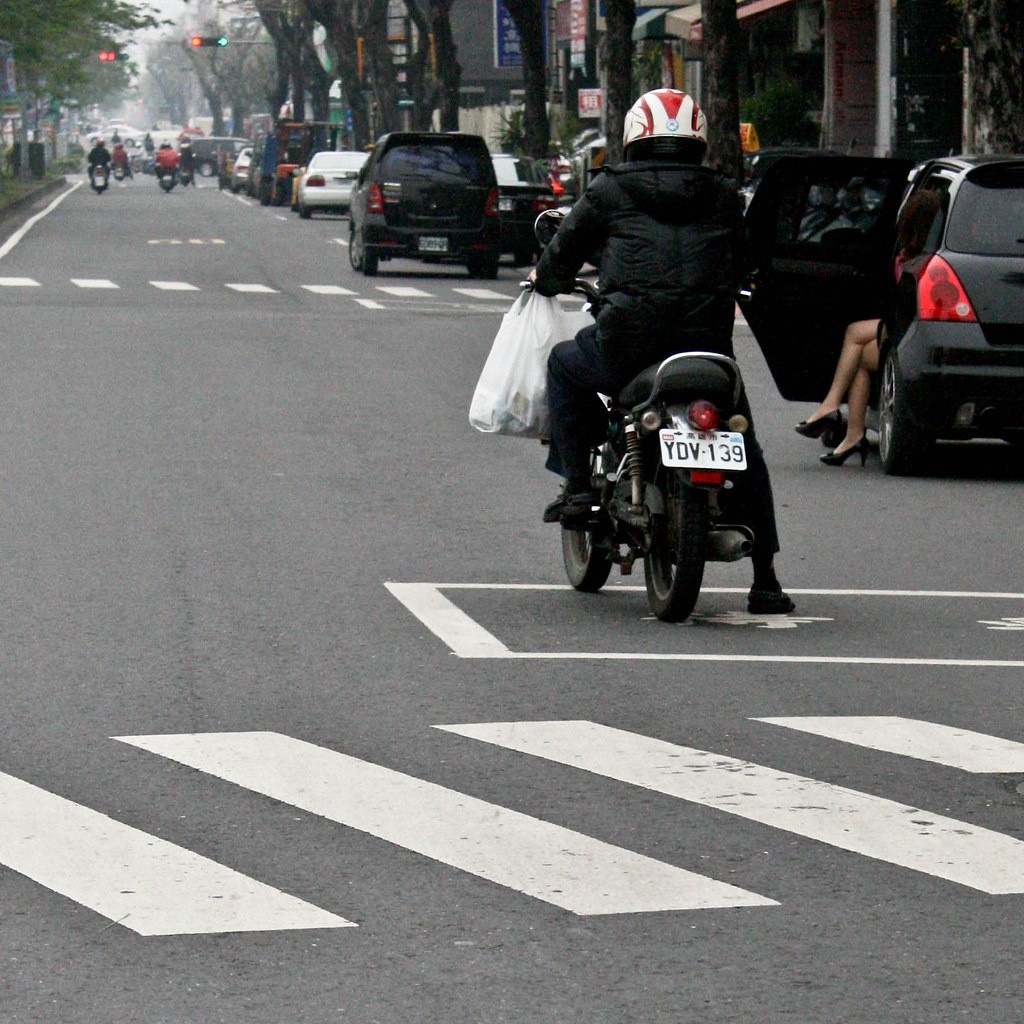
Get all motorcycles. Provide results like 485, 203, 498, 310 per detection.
155, 162, 178, 192
91, 164, 107, 195
113, 160, 125, 182
519, 204, 760, 627
178, 153, 196, 188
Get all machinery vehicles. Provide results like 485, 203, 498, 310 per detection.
262, 117, 348, 207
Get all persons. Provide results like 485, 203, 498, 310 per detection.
796, 191, 941, 467
87, 131, 199, 190
527, 88, 796, 614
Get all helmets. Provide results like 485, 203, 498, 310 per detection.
115, 143, 121, 150
162, 141, 170, 148
96, 136, 105, 145
621, 89, 708, 151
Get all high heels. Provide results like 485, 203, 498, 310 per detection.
819, 437, 870, 467
794, 408, 842, 439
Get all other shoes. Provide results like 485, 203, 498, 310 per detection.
543, 486, 595, 526
748, 581, 796, 615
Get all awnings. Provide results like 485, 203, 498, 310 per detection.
594, 0, 790, 43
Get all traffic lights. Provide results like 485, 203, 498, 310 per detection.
193, 37, 228, 47
99, 52, 129, 61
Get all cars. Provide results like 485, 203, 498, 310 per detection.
87, 125, 145, 149
146, 124, 183, 140
296, 150, 371, 221
734, 147, 1024, 472
222, 143, 255, 192
488, 154, 561, 269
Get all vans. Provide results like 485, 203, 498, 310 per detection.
179, 137, 252, 178
345, 133, 498, 280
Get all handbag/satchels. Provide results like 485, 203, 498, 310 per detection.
470, 288, 611, 446
